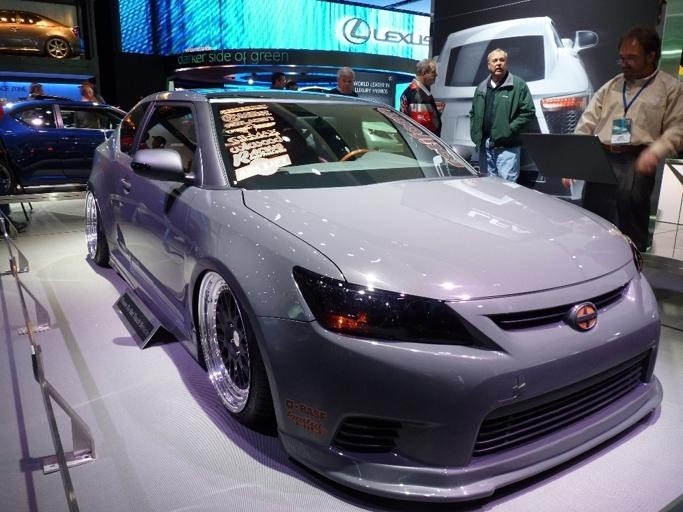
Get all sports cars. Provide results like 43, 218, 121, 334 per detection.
85, 89, 663, 502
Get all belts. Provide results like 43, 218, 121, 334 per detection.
603, 145, 648, 153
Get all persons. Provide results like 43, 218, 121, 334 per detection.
80, 85, 98, 102
26, 82, 44, 100
561, 25, 683, 252
399, 59, 443, 138
325, 67, 358, 97
468, 48, 536, 183
269, 72, 285, 90
285, 79, 298, 91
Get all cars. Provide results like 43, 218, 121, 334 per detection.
0, 9, 80, 59
1, 99, 146, 195
423, 15, 599, 158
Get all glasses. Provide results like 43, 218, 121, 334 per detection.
615, 55, 645, 64
